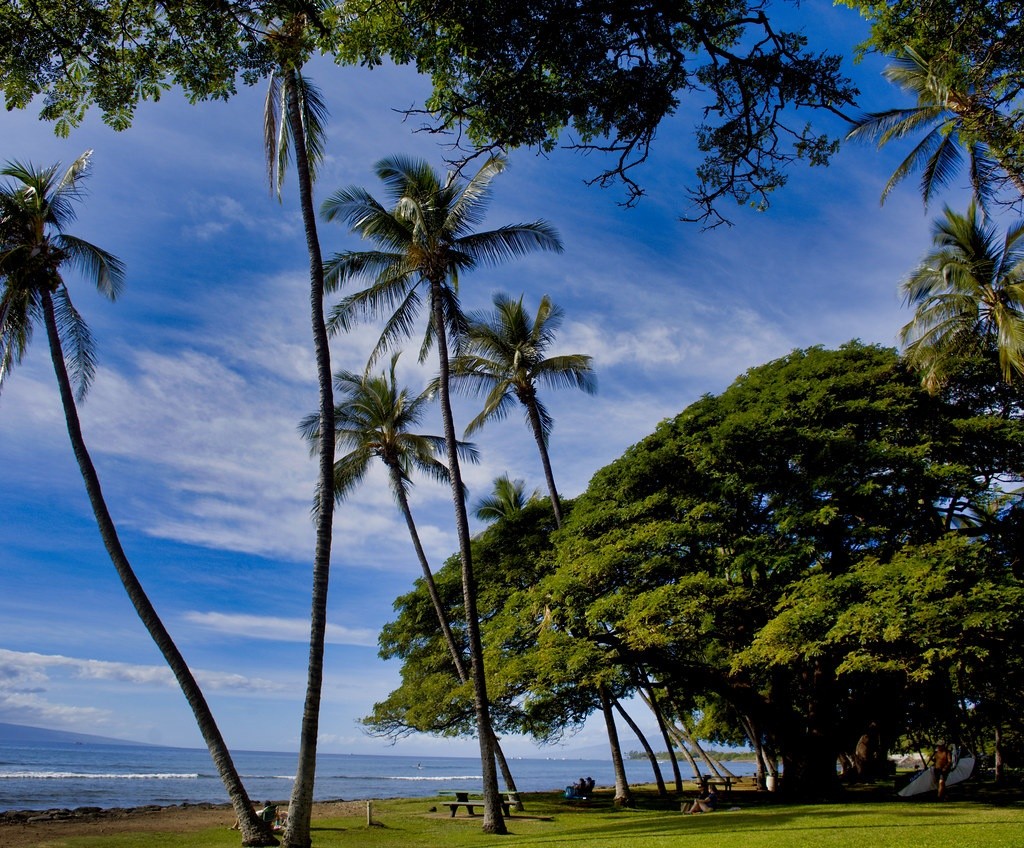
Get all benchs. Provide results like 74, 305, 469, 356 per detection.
440, 799, 519, 817
695, 781, 737, 795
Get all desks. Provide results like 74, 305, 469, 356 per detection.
691, 776, 742, 795
439, 790, 525, 817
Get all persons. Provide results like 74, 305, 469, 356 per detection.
572, 777, 592, 789
685, 784, 719, 813
929, 740, 951, 800
229, 800, 287, 829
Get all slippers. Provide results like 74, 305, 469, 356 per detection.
228, 828, 240, 830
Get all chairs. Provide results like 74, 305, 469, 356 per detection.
257, 804, 279, 831
566, 780, 595, 800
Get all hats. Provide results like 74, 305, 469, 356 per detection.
265, 801, 271, 807
586, 777, 592, 781
935, 739, 945, 745
